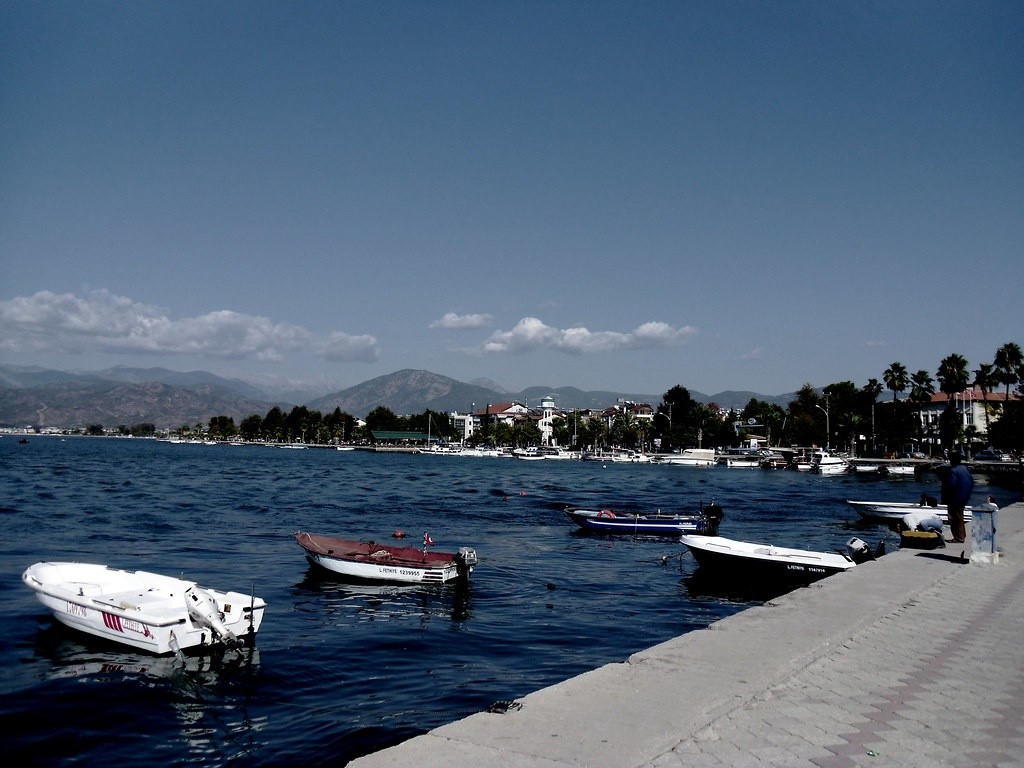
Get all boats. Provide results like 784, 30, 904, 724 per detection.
856, 465, 879, 473
847, 499, 973, 524
337, 447, 355, 451
887, 463, 915, 476
513, 446, 578, 461
818, 457, 849, 475
295, 531, 478, 585
587, 443, 656, 462
797, 450, 830, 472
680, 535, 885, 598
727, 459, 759, 467
171, 439, 218, 446
650, 447, 717, 467
229, 443, 246, 446
761, 455, 787, 470
280, 445, 309, 450
418, 444, 513, 458
22, 562, 267, 654
564, 499, 709, 535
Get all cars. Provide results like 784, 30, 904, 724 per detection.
898, 452, 926, 459
974, 450, 1003, 461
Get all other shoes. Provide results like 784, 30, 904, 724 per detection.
949, 538, 965, 543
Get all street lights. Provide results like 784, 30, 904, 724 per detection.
815, 404, 830, 448
659, 412, 672, 429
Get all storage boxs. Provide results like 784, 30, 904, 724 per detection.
901, 531, 938, 550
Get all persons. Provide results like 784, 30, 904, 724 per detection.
919, 493, 937, 507
888, 512, 946, 548
941, 453, 974, 542
943, 449, 950, 462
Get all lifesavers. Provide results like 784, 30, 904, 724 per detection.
597, 509, 614, 518
